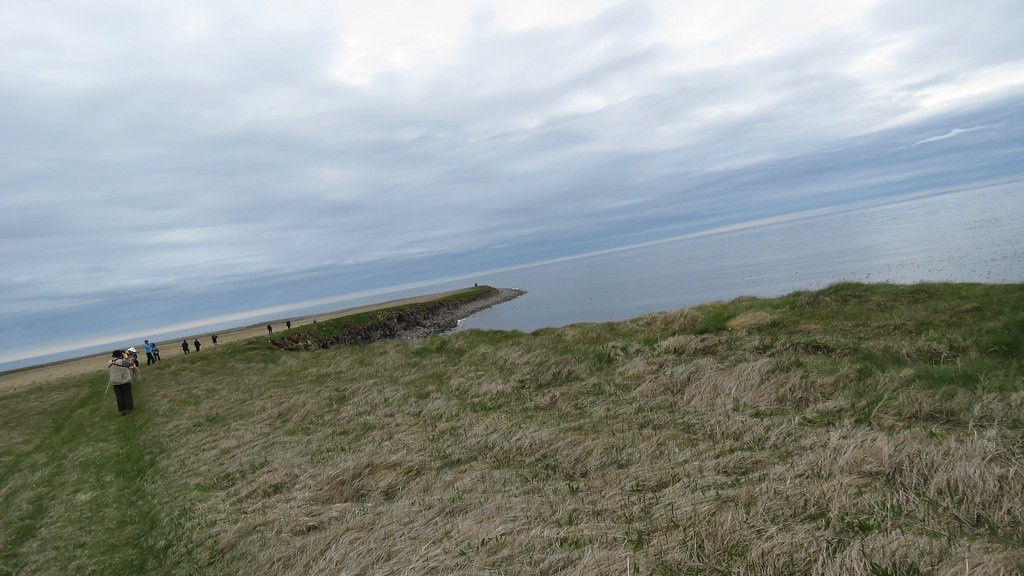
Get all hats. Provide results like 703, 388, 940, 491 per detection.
128, 348, 137, 353
112, 350, 122, 357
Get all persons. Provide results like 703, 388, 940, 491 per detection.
106, 350, 134, 417
150, 342, 160, 362
181, 340, 189, 354
211, 334, 218, 347
266, 325, 272, 335
285, 320, 291, 330
143, 340, 155, 366
193, 338, 201, 352
120, 347, 139, 378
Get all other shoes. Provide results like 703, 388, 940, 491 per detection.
121, 410, 129, 416
